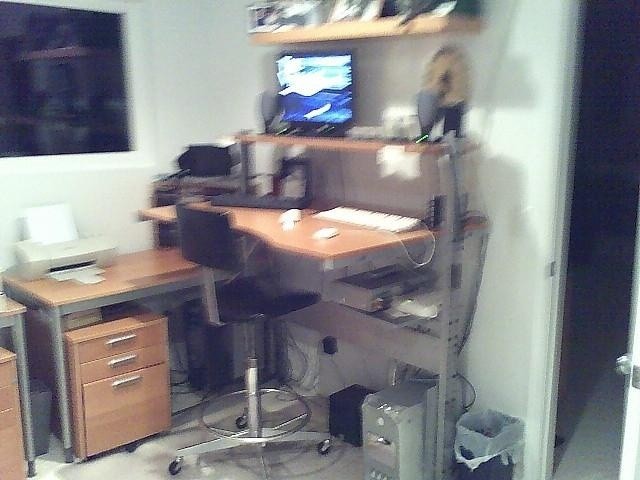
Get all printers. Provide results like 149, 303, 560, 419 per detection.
16, 211, 116, 285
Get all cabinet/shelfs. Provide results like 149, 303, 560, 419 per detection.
0, 344, 26, 480
232, 11, 486, 157
26, 310, 173, 461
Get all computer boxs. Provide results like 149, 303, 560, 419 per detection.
361, 377, 465, 480
182, 300, 284, 399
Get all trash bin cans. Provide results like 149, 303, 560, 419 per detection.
454, 409, 523, 479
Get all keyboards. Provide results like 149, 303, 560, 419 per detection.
315, 207, 422, 234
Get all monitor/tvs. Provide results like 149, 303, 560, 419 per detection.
278, 52, 354, 139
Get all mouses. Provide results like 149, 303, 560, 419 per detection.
314, 229, 337, 240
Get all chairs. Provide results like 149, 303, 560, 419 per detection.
170, 205, 334, 480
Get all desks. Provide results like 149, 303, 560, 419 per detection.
1, 246, 278, 463
139, 199, 490, 479
0, 293, 30, 480
